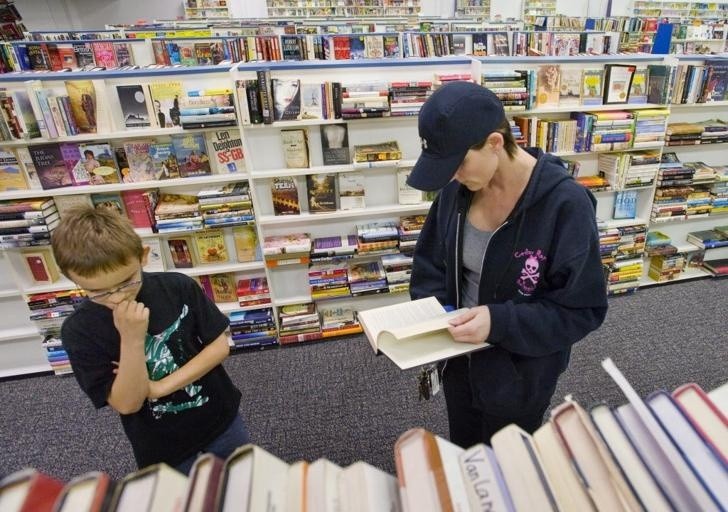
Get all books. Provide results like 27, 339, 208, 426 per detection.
1, 356, 727, 511
2, 3, 727, 383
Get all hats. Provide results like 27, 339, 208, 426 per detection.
402, 82, 507, 192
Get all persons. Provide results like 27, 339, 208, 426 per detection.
405, 78, 610, 451
46, 205, 256, 480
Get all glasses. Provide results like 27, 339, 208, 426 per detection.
72, 276, 144, 302
